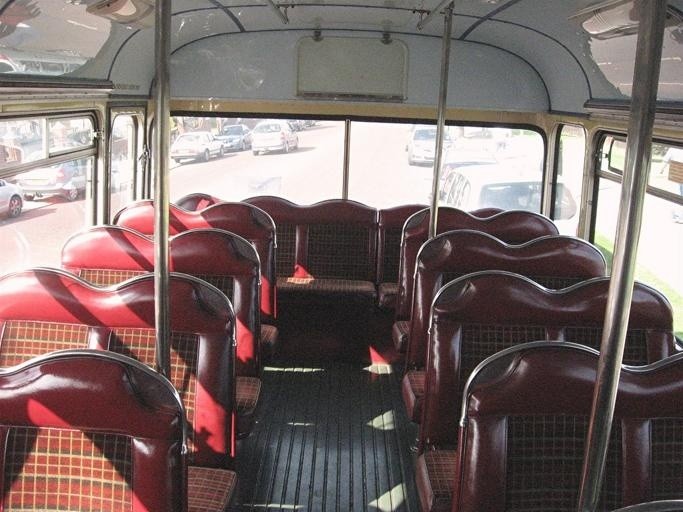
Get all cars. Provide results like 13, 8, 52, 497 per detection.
451, 124, 508, 138
254, 120, 298, 152
29, 136, 91, 159
0, 176, 26, 219
292, 119, 309, 129
170, 130, 223, 161
23, 161, 90, 200
403, 125, 451, 161
450, 135, 510, 168
440, 161, 581, 234
219, 125, 252, 150
